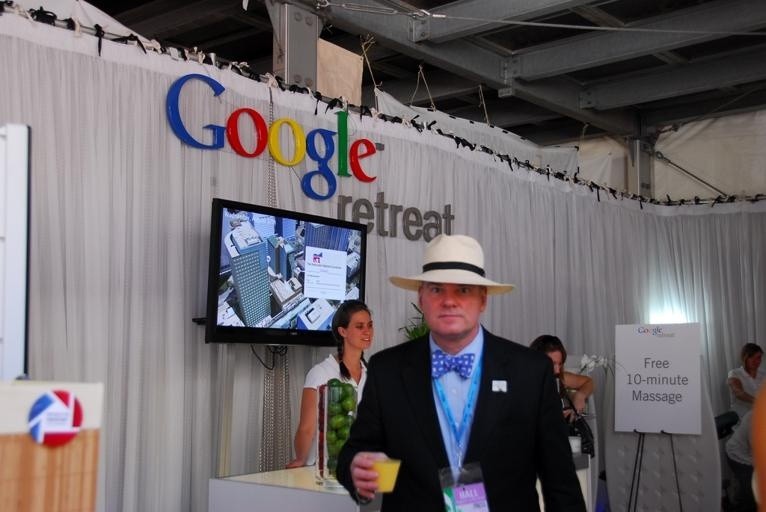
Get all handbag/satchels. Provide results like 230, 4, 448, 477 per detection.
559, 378, 594, 459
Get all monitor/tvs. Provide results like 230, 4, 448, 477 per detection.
205, 198, 367, 346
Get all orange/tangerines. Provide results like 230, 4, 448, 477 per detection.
326, 378, 355, 478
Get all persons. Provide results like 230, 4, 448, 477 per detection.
335, 234, 588, 511
724, 409, 760, 512
286, 300, 374, 469
728, 343, 765, 424
528, 335, 595, 423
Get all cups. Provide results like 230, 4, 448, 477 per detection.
569, 436, 582, 453
366, 459, 402, 494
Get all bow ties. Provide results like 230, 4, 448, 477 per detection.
431, 349, 475, 380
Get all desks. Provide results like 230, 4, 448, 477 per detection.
208, 465, 383, 512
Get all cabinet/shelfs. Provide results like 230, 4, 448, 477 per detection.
627, 429, 683, 512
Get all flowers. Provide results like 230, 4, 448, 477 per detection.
567, 354, 614, 390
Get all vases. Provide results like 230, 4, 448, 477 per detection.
562, 391, 576, 410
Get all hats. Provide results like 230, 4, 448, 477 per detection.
389, 234, 515, 295
